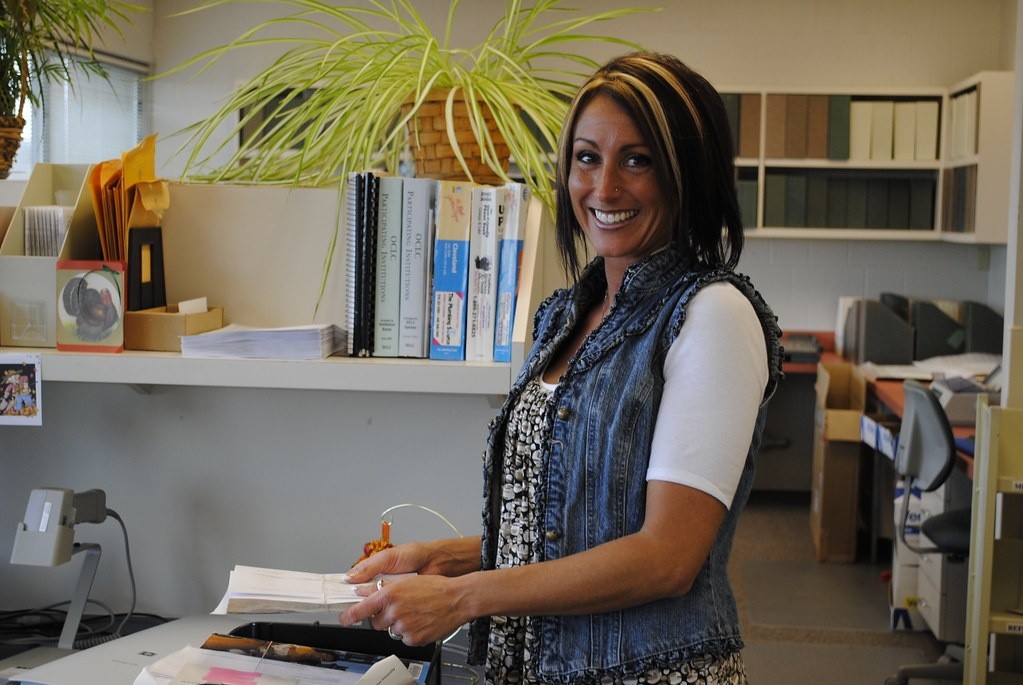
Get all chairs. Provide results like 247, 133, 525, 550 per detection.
894, 378, 973, 562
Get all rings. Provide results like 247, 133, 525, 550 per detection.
388, 625, 403, 640
377, 580, 383, 590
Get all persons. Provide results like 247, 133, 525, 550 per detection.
341, 53, 786, 685
4, 375, 34, 412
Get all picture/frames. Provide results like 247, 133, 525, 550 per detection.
0, 353, 43, 427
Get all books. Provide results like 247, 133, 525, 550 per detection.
24, 206, 74, 256
345, 171, 532, 362
225, 569, 417, 615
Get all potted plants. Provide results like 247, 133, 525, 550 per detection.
142, 0, 665, 326
0, 0, 155, 182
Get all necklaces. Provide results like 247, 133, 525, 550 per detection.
601, 290, 607, 322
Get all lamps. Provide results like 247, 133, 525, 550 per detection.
0, 485, 137, 685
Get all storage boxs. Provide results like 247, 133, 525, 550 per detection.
860, 412, 899, 452
714, 90, 978, 231
876, 420, 902, 461
838, 296, 914, 366
54, 258, 130, 354
0, 162, 92, 347
243, 88, 333, 151
881, 292, 966, 361
961, 301, 1005, 356
810, 359, 866, 566
125, 306, 224, 352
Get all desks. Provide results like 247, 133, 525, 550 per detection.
779, 330, 975, 564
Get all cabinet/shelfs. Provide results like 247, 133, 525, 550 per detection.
941, 71, 1015, 244
959, 393, 1023, 685
238, 70, 950, 249
0, 179, 569, 685
915, 470, 974, 646
